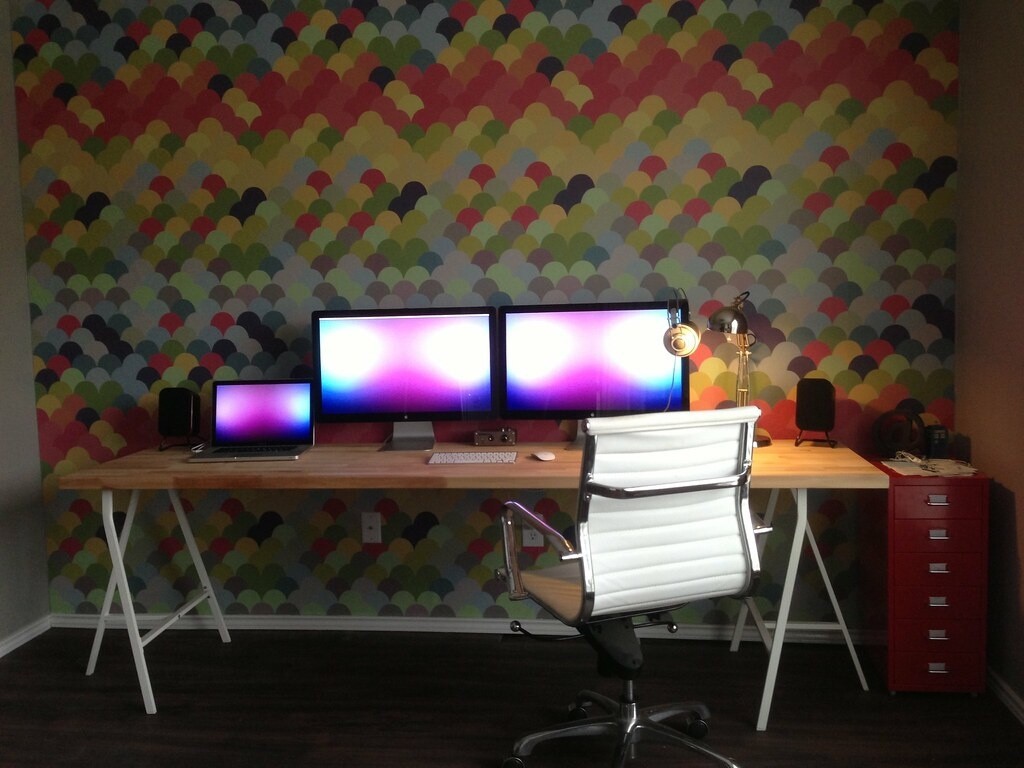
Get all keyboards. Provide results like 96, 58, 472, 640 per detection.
426, 451, 518, 464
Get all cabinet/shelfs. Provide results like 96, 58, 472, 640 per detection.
856, 456, 991, 706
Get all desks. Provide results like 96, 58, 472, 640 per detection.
60, 443, 890, 734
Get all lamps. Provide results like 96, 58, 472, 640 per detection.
706, 291, 771, 447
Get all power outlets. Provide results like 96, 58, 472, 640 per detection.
361, 512, 381, 544
522, 513, 545, 548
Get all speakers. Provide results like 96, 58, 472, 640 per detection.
794, 377, 837, 448
157, 388, 201, 451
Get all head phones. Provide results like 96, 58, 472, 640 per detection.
664, 287, 702, 357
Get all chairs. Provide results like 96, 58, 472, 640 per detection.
493, 406, 767, 768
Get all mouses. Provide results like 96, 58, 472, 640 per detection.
531, 452, 555, 461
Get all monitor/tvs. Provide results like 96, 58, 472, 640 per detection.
311, 298, 691, 453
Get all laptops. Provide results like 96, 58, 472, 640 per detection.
186, 379, 313, 461
